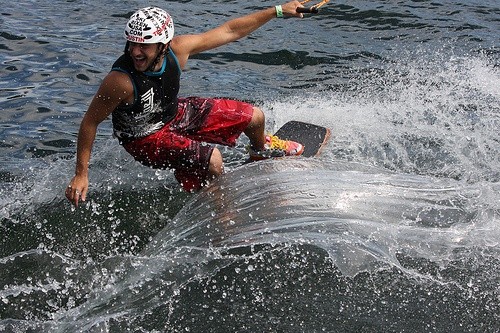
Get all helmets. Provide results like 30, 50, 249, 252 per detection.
123, 6, 174, 44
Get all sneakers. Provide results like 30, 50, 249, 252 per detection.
248, 133, 304, 161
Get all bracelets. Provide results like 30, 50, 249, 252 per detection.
275, 5, 283, 17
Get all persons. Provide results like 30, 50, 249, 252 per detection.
66, 0, 304, 225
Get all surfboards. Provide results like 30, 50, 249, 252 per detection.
244, 120, 332, 167
